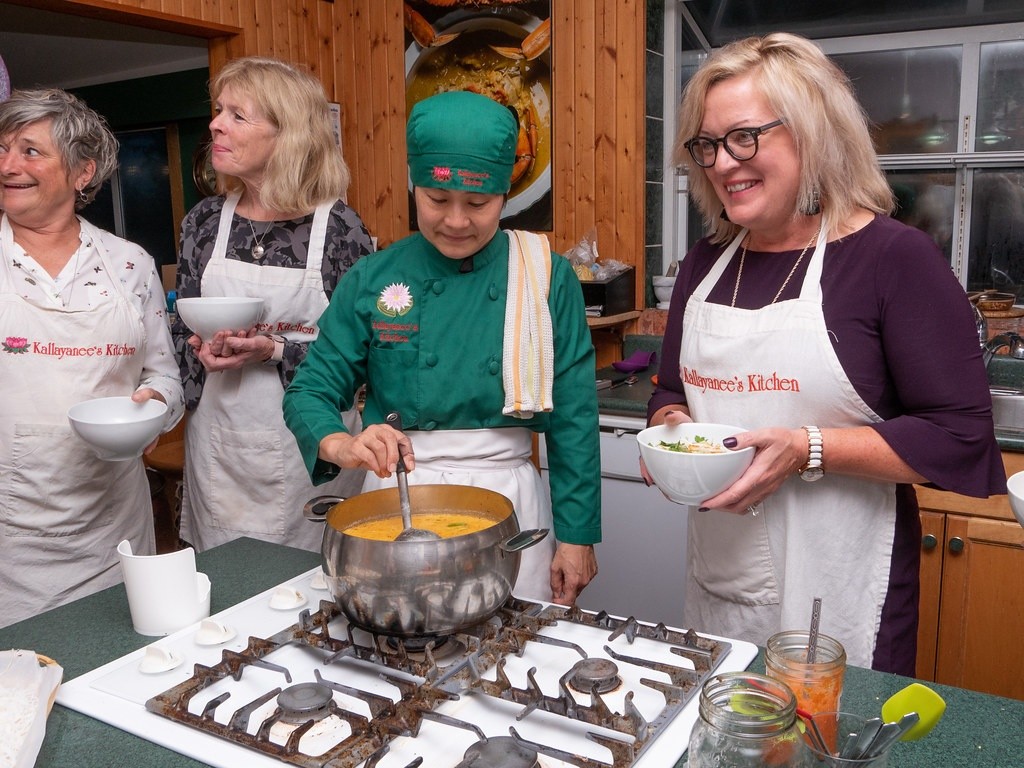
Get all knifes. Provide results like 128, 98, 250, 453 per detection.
594, 375, 632, 390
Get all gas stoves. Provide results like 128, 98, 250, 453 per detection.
55, 563, 759, 768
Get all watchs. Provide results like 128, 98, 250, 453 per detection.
798, 426, 825, 482
264, 334, 284, 367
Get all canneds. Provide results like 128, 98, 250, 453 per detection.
762, 631, 846, 768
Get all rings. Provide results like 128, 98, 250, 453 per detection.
748, 505, 759, 517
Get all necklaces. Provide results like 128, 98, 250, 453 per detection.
731, 225, 821, 307
245, 192, 279, 259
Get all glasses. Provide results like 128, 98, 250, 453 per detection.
683, 116, 788, 168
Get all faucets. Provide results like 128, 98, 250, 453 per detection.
980, 331, 1024, 367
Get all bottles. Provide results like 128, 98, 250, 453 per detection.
764, 630, 846, 761
688, 672, 814, 768
167, 290, 176, 313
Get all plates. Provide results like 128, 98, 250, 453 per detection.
651, 374, 657, 384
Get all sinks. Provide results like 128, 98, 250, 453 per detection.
990, 394, 1023, 431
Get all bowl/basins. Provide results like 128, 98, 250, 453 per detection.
177, 297, 265, 343
636, 422, 756, 506
406, 5, 550, 221
68, 397, 168, 461
1006, 471, 1024, 529
966, 291, 1015, 310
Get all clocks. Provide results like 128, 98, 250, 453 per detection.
192, 142, 218, 197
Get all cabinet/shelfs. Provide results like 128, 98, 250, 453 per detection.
913, 448, 1022, 702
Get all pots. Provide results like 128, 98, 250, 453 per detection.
303, 484, 550, 637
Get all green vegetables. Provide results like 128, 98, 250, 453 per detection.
661, 435, 708, 454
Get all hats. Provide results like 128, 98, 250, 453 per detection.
405, 91, 517, 194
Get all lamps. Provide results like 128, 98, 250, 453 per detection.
913, 112, 949, 146
975, 115, 1011, 144
899, 51, 912, 119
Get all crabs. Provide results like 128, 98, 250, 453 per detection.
401, 0, 552, 184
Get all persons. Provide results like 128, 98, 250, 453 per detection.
906, 170, 1024, 291
637, 32, 1009, 678
0, 85, 187, 627
283, 91, 604, 606
165, 57, 375, 552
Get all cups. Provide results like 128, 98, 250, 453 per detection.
795, 712, 889, 768
